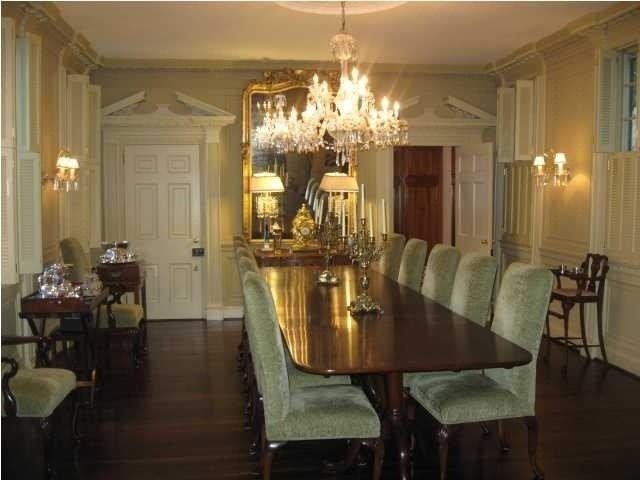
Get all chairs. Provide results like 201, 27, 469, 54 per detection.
303, 178, 329, 230
546, 253, 610, 373
60, 236, 150, 394
1, 335, 79, 480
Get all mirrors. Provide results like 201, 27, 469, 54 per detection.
240, 76, 357, 252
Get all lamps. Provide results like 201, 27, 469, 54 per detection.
532, 151, 570, 176
250, 175, 286, 254
319, 172, 361, 244
249, 91, 321, 157
319, 173, 333, 225
300, 1, 410, 166
41, 155, 80, 194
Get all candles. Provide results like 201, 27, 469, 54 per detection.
342, 203, 346, 236
382, 198, 387, 233
361, 184, 365, 218
369, 206, 374, 236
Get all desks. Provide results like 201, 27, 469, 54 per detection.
19, 281, 109, 403
106, 276, 147, 320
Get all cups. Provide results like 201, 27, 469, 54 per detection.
559, 263, 567, 274
572, 267, 584, 274
